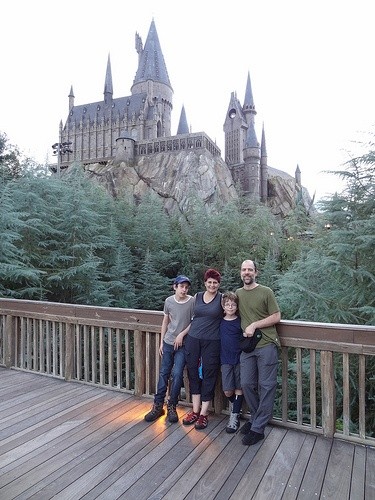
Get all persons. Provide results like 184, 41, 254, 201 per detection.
220, 292, 247, 434
184, 269, 223, 430
144, 274, 197, 424
234, 259, 282, 446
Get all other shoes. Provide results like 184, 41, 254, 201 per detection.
226, 411, 240, 433
167, 402, 178, 422
144, 403, 164, 422
240, 421, 252, 434
230, 401, 242, 418
241, 430, 265, 444
195, 415, 208, 429
182, 408, 201, 425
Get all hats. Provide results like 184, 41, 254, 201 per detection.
239, 328, 262, 353
174, 275, 191, 286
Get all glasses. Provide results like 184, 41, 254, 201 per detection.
224, 304, 237, 308
